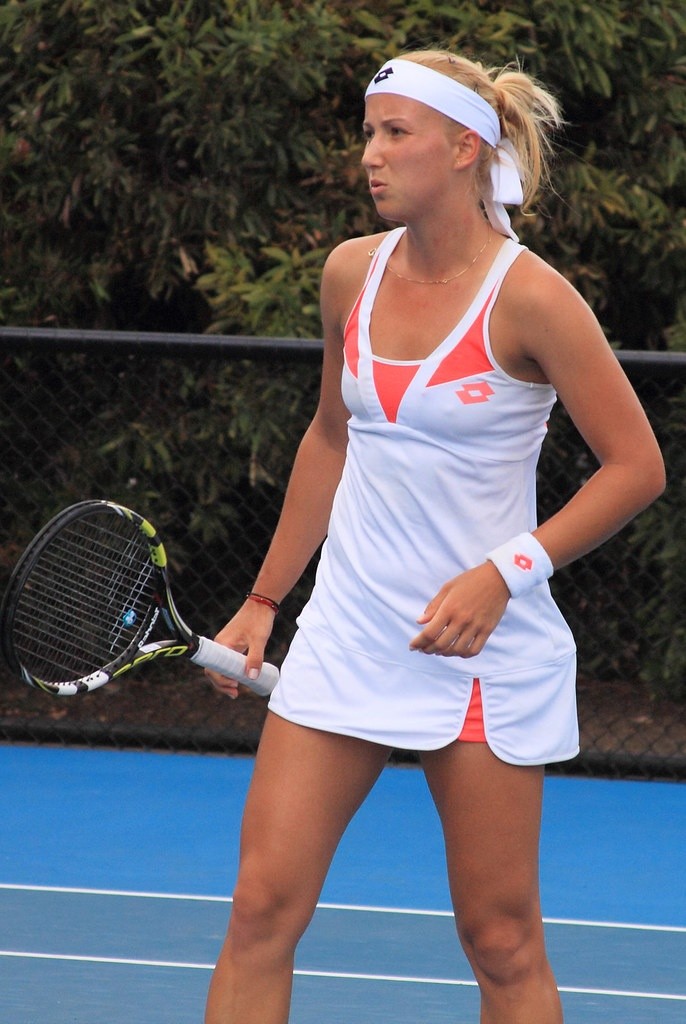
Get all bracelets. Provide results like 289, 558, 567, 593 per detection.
488, 533, 557, 600
250, 591, 282, 613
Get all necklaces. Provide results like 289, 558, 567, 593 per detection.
369, 232, 495, 284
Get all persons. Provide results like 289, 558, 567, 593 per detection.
202, 50, 668, 1024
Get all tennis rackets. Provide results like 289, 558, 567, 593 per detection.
0, 497, 278, 702
